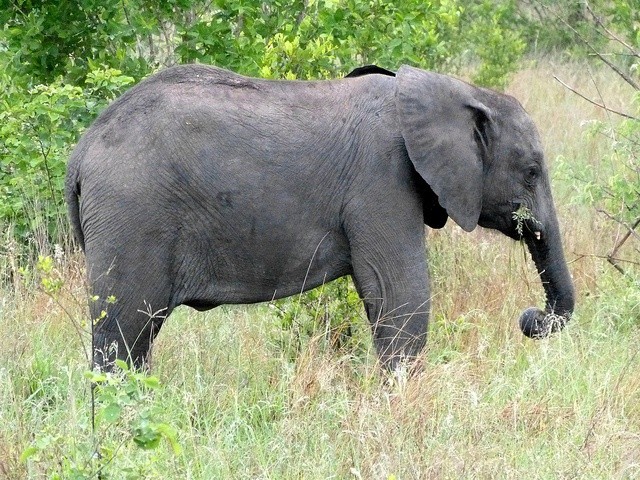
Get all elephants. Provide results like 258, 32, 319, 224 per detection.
62, 62, 577, 391
